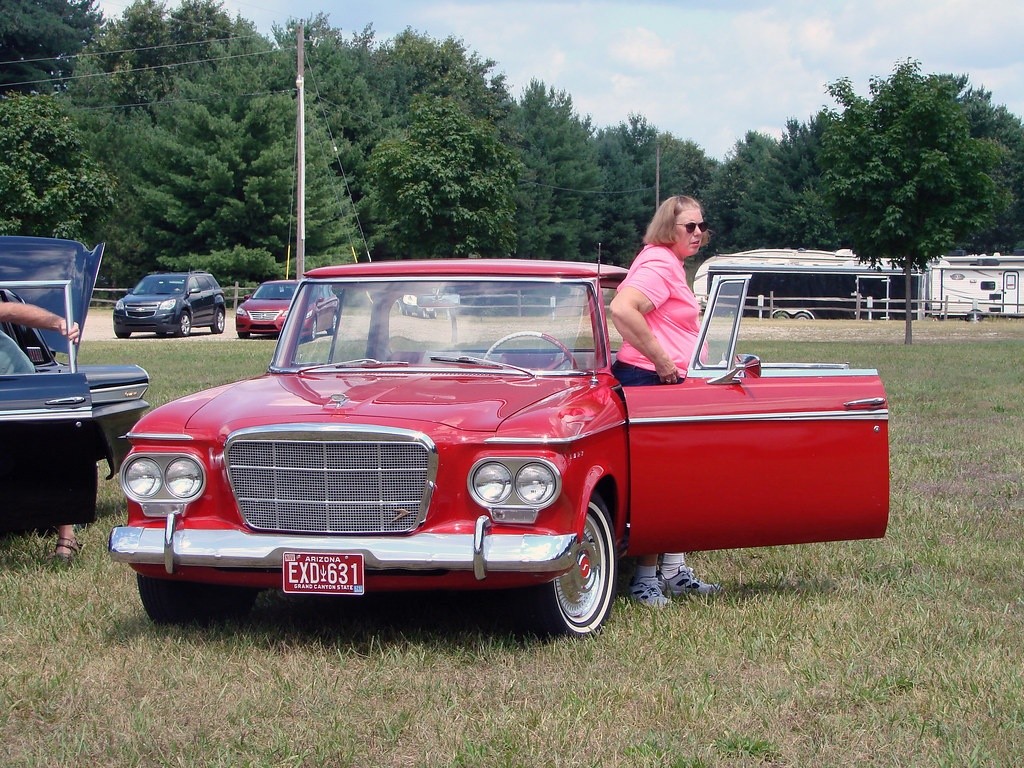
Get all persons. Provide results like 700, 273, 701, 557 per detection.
2, 297, 83, 564
608, 195, 718, 612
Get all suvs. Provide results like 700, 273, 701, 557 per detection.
113, 270, 227, 339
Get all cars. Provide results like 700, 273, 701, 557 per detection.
235, 279, 340, 342
107, 256, 891, 637
1, 236, 153, 533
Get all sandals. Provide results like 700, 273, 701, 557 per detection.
50, 537, 83, 570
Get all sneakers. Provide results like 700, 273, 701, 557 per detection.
657, 564, 722, 596
628, 576, 675, 610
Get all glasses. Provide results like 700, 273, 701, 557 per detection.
676, 222, 709, 233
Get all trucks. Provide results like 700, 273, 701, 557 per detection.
924, 255, 1024, 321
694, 247, 929, 323
